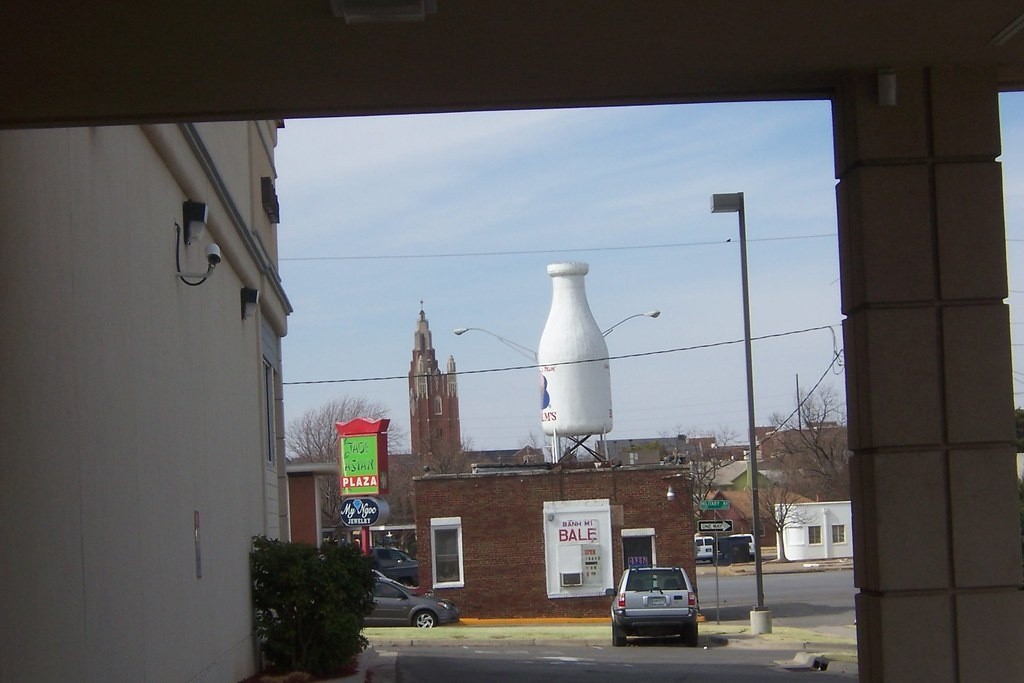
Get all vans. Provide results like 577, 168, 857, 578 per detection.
728, 534, 756, 561
695, 534, 714, 564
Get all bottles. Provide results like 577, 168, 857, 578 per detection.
536, 261, 613, 437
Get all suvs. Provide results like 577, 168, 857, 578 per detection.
605, 566, 700, 647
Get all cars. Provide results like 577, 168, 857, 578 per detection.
370, 547, 417, 562
362, 578, 460, 629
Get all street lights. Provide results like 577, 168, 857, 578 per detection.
710, 191, 773, 637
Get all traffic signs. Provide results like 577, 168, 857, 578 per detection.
697, 519, 733, 533
